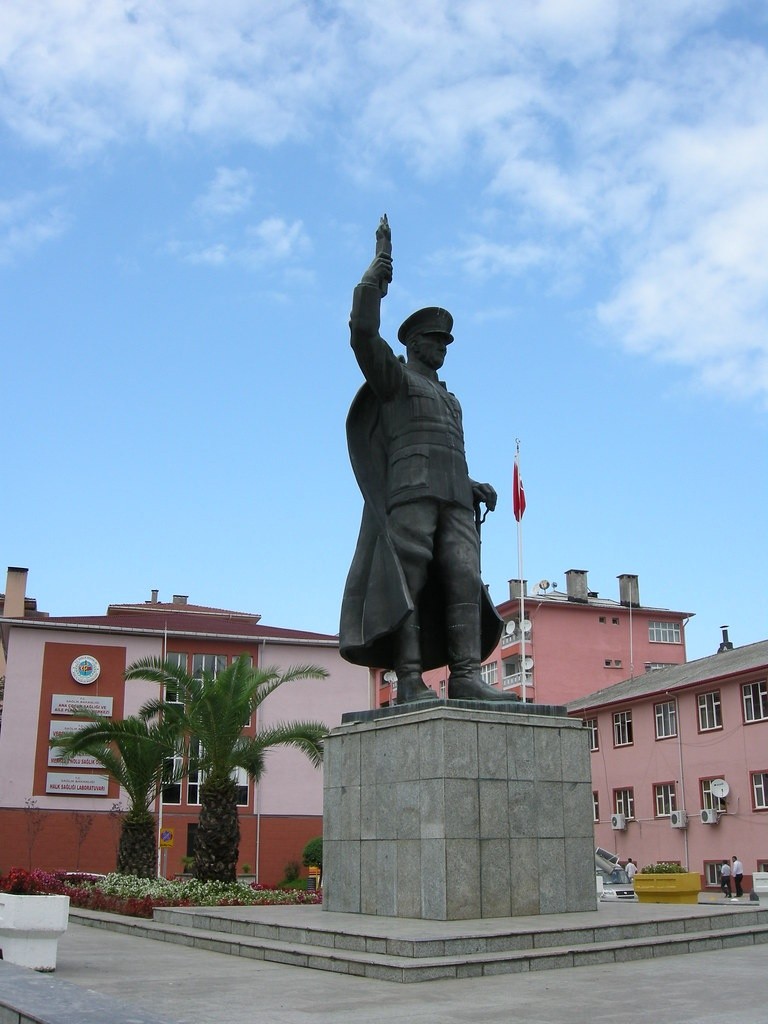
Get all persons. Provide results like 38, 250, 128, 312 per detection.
625, 855, 744, 898
337, 211, 518, 701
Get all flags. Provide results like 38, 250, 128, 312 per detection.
512, 445, 526, 521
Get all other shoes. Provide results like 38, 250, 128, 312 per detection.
728, 895, 732, 898
726, 894, 728, 896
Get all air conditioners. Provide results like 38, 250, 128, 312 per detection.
611, 813, 625, 830
670, 810, 687, 828
700, 809, 718, 824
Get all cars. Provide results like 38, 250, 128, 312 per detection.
595, 863, 637, 901
